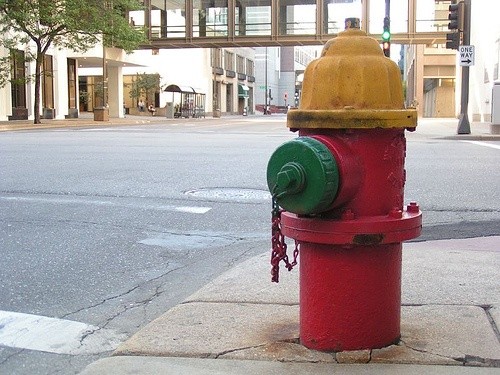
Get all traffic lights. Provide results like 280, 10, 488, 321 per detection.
445, 2, 463, 50
382, 16, 392, 57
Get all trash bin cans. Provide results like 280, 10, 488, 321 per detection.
94, 107, 109, 121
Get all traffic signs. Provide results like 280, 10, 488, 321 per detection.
458, 42, 477, 67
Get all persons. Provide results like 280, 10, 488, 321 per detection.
138, 99, 144, 112
148, 104, 159, 117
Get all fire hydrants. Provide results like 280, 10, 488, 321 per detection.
264, 15, 421, 355
